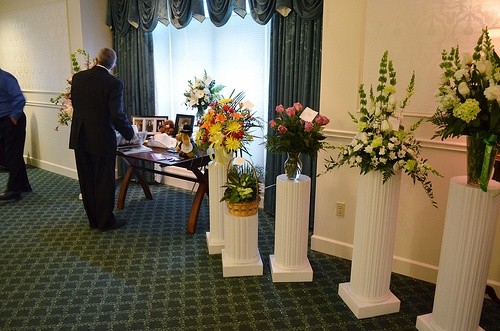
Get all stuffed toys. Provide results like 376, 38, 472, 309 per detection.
160, 120, 175, 135
175, 125, 197, 159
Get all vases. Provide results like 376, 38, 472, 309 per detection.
196, 104, 207, 120
284, 149, 301, 181
217, 147, 232, 163
465, 137, 498, 187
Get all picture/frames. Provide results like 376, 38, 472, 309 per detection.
133, 117, 167, 135
176, 114, 195, 131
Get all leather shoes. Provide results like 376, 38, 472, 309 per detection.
91, 219, 126, 232
19, 182, 32, 192
0, 189, 21, 201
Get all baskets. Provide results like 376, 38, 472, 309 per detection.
224, 157, 260, 217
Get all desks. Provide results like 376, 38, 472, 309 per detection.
117, 143, 215, 234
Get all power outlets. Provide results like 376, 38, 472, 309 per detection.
336, 202, 345, 217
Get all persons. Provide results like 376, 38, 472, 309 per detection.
0, 68, 32, 203
68, 48, 138, 232
135, 120, 164, 132
183, 121, 190, 130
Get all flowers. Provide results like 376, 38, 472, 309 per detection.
318, 50, 445, 208
219, 157, 263, 204
259, 102, 329, 154
183, 69, 227, 109
49, 48, 120, 131
195, 89, 268, 158
424, 26, 500, 142
